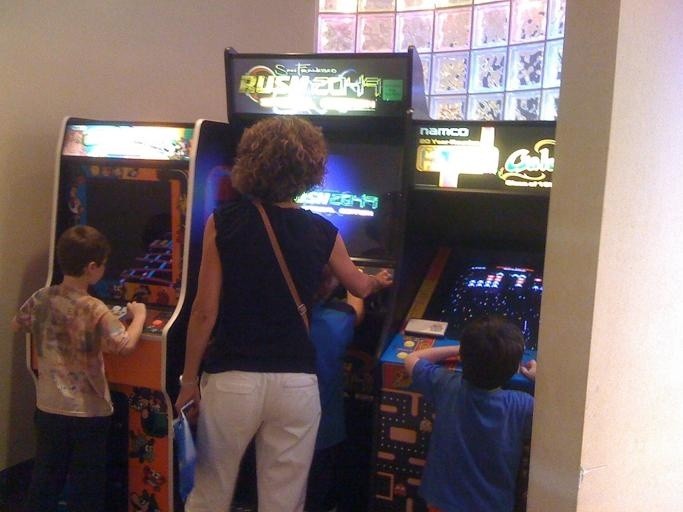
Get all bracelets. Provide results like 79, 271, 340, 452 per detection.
180, 375, 198, 386
369, 273, 379, 288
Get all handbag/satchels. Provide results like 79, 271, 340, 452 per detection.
173, 399, 197, 502
251, 199, 311, 347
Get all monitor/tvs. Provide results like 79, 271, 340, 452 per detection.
241, 139, 406, 266
424, 262, 547, 354
113, 216, 172, 286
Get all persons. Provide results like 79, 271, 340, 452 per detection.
404, 313, 538, 512
14, 225, 146, 512
302, 254, 367, 511
176, 119, 392, 512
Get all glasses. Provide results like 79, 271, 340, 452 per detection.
100, 261, 110, 272
